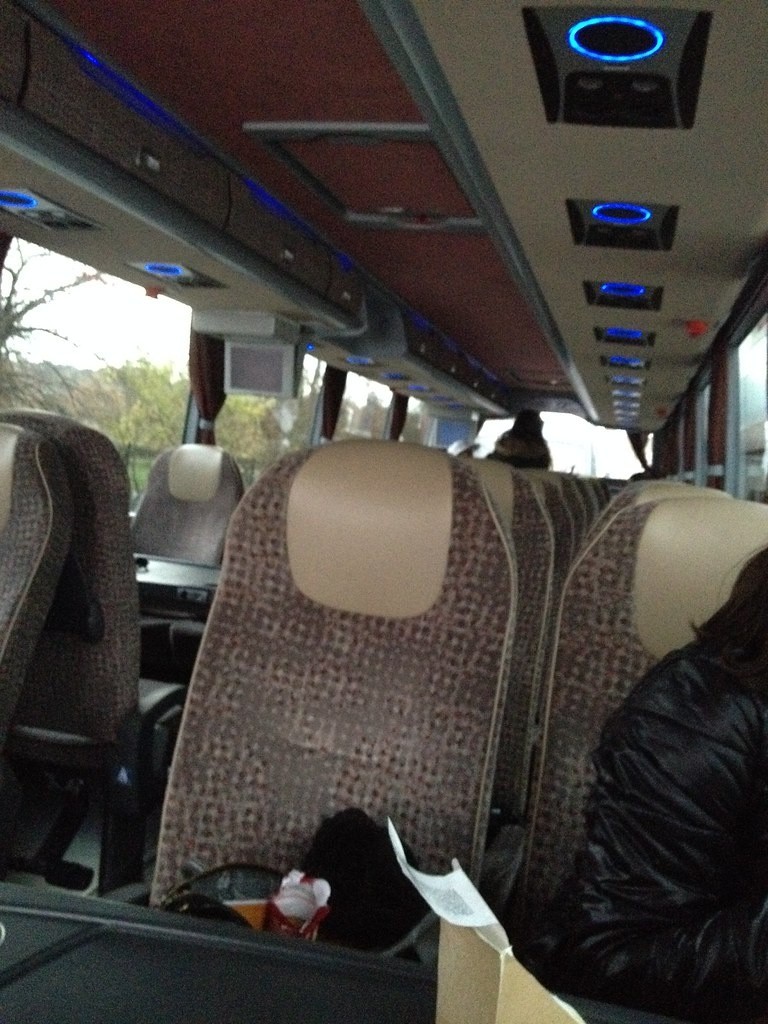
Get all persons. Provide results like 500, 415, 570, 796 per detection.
446, 439, 480, 461
486, 408, 552, 471
547, 544, 768, 1024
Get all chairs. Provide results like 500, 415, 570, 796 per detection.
0, 411, 768, 968
